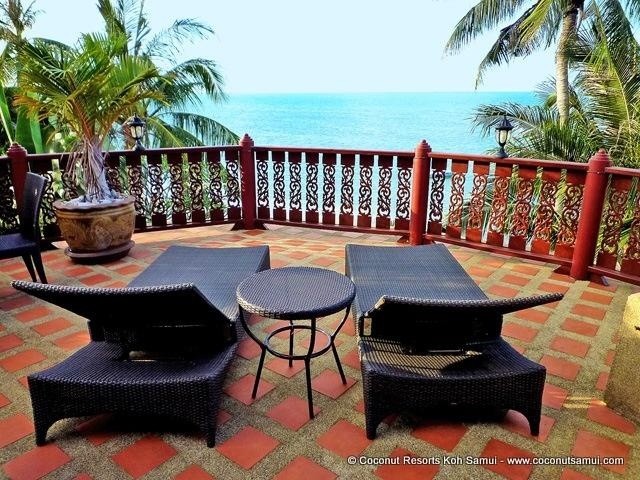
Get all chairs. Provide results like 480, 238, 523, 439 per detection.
344, 244, 565, 440
0, 172, 48, 284
10, 246, 271, 449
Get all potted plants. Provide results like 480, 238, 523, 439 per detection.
0, 0, 223, 264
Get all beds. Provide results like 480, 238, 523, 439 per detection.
236, 266, 356, 419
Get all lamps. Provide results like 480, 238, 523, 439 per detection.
126, 112, 147, 151
493, 113, 514, 158
